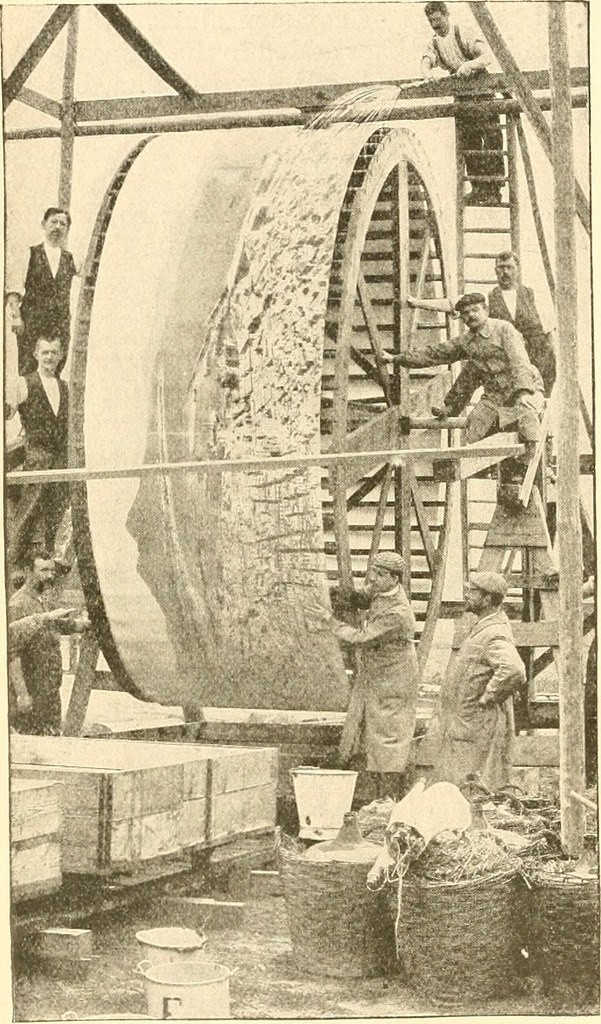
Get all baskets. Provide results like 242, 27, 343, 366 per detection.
274, 782, 600, 1006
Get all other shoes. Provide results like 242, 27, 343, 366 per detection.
434, 461, 455, 482
504, 459, 528, 484
432, 404, 454, 420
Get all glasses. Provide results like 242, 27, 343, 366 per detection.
469, 587, 484, 592
372, 568, 388, 576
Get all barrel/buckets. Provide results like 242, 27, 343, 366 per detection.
134, 927, 205, 972
290, 769, 358, 840
136, 959, 240, 1018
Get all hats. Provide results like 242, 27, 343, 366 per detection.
372, 552, 406, 573
455, 293, 485, 310
470, 572, 508, 595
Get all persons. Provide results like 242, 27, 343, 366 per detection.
405, 250, 555, 417
422, 571, 527, 802
4, 207, 85, 377
6, 607, 77, 665
5, 334, 70, 568
7, 547, 90, 737
306, 551, 421, 803
416, 2, 506, 207
379, 292, 546, 464
